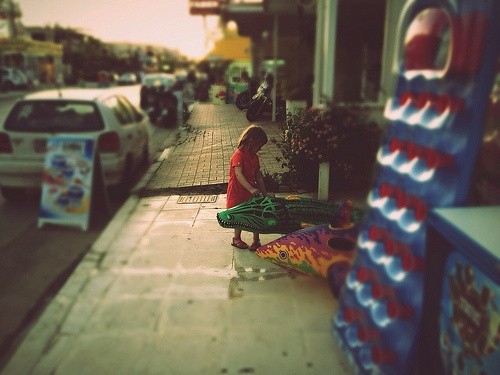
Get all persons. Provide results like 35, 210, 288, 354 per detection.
97, 70, 110, 88
226, 125, 275, 252
187, 65, 211, 101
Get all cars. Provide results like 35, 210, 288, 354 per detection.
118, 72, 137, 85
0, 88, 155, 207
1, 65, 28, 90
139, 73, 178, 111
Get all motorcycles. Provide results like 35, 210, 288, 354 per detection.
246, 73, 313, 122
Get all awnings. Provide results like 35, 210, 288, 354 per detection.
201, 34, 253, 61
